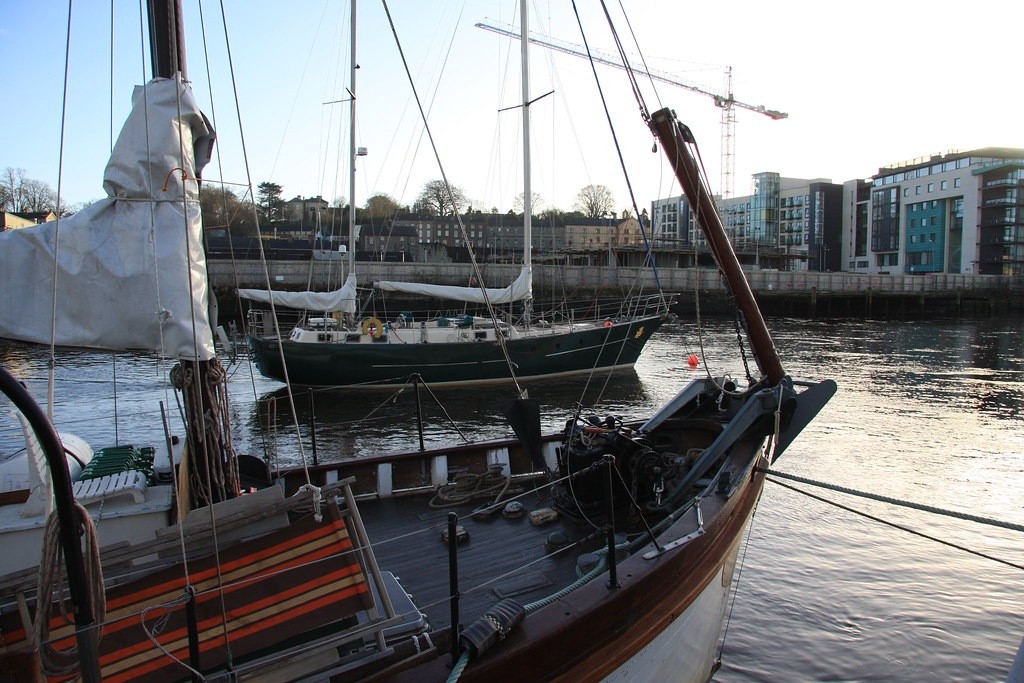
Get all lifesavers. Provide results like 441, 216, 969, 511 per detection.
362, 318, 383, 338
224, 455, 272, 490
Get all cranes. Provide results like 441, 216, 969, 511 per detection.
472, 16, 789, 201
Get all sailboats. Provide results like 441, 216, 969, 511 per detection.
0, 3, 838, 683
